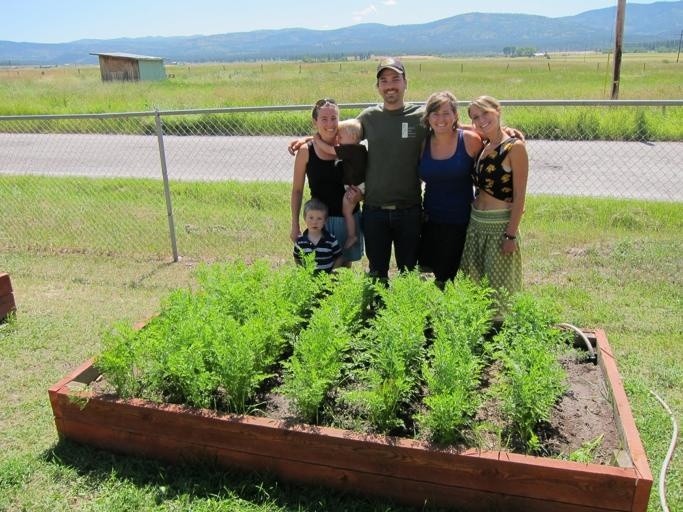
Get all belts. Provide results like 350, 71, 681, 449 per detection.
365, 204, 421, 211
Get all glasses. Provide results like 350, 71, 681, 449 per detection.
315, 99, 337, 109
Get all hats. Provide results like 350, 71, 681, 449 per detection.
376, 56, 405, 78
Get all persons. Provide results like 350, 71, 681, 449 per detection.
289, 98, 363, 269
462, 94, 528, 320
293, 200, 344, 277
312, 119, 368, 250
419, 92, 484, 293
288, 58, 526, 311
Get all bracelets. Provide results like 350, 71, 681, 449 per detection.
504, 233, 516, 241
